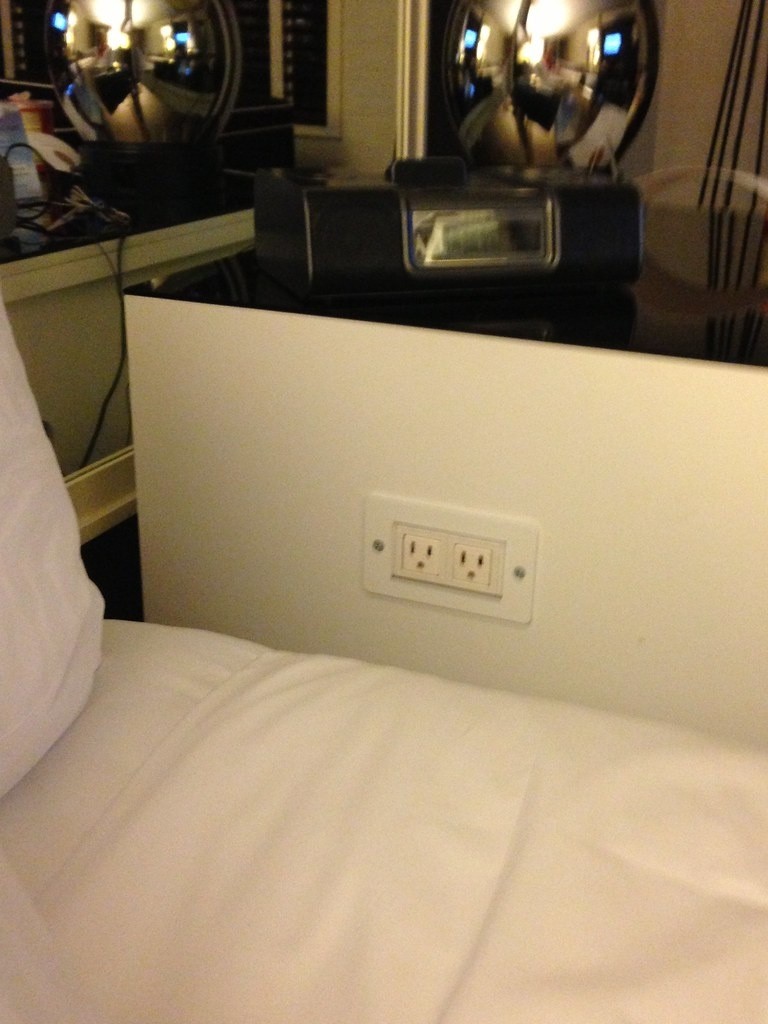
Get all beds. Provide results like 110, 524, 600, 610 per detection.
1, 618, 766, 1023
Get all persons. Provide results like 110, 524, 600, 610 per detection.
86, 25, 185, 94
505, 34, 595, 159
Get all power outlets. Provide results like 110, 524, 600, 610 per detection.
360, 494, 541, 627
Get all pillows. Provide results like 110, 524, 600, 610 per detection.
0, 295, 105, 797
